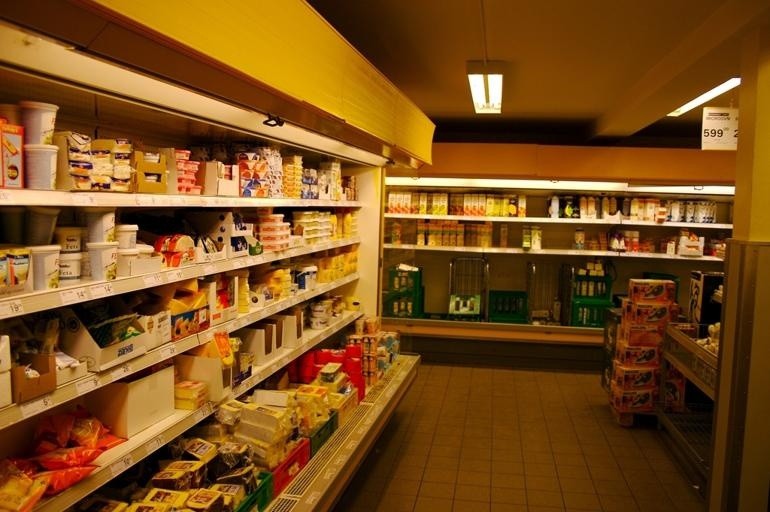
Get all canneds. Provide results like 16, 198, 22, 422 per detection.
575, 230, 585, 249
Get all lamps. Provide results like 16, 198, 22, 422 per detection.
667, 78, 740, 117
468, 72, 504, 114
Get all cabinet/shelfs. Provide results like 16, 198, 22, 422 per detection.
653, 292, 726, 506
418, 177, 736, 363
0, 35, 328, 512
330, 139, 420, 508
